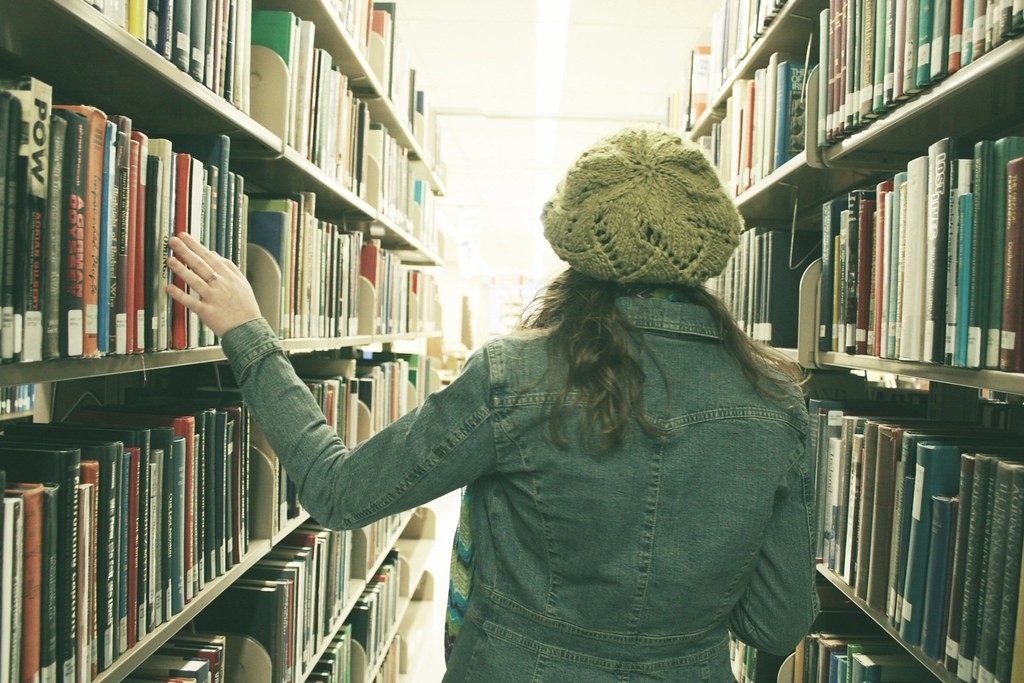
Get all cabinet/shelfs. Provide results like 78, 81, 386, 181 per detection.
686, 0, 1024, 683
0, 0, 446, 683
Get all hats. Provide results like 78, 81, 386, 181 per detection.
539, 127, 740, 287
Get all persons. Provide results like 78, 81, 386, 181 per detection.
165, 127, 821, 683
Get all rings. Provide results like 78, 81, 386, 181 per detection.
206, 272, 218, 283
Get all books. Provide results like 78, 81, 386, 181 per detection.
0, 1, 452, 683
668, 1, 1024, 683
